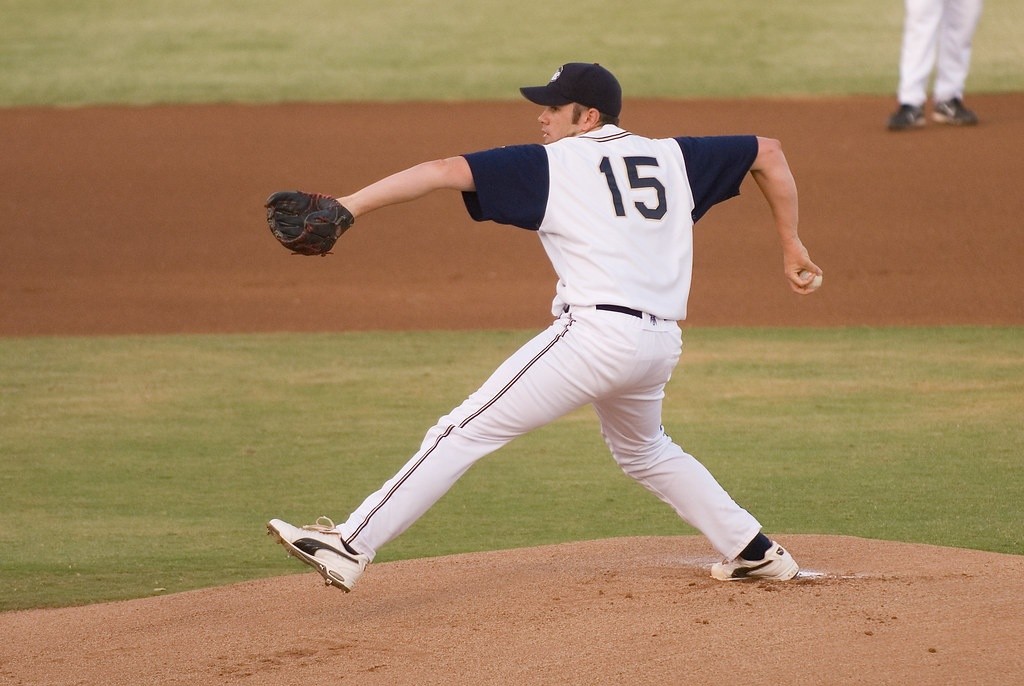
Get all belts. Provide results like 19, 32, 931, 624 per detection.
564, 304, 668, 321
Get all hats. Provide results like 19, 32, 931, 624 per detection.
519, 62, 623, 118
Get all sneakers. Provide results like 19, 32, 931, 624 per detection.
266, 515, 370, 594
710, 540, 800, 582
931, 97, 977, 127
886, 102, 927, 131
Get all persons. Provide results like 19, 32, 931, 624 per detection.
886, 0, 983, 129
263, 61, 823, 593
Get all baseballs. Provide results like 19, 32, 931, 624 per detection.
800, 270, 823, 291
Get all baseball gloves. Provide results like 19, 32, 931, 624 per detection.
264, 191, 355, 256
